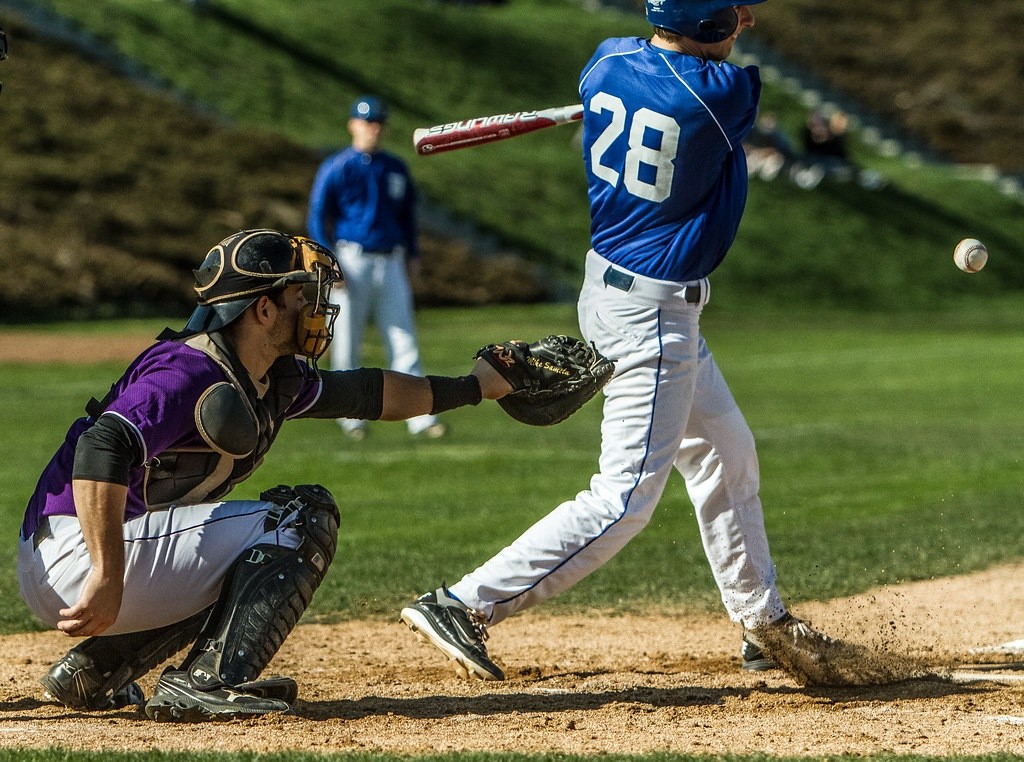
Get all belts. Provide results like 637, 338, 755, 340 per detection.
28, 516, 52, 552
600, 267, 701, 302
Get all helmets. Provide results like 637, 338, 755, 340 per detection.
350, 94, 389, 125
645, 0, 768, 44
185, 227, 344, 387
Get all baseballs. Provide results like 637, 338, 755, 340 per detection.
952, 238, 991, 273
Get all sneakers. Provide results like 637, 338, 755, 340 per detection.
741, 609, 871, 668
37, 648, 144, 712
398, 580, 505, 682
142, 665, 297, 722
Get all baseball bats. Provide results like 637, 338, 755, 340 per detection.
412, 103, 584, 156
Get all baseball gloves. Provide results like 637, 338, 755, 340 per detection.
473, 334, 616, 428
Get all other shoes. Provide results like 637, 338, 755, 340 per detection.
347, 426, 371, 441
427, 421, 448, 437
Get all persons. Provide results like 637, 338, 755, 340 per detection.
398, 1, 873, 681
305, 97, 447, 437
19, 229, 530, 724
